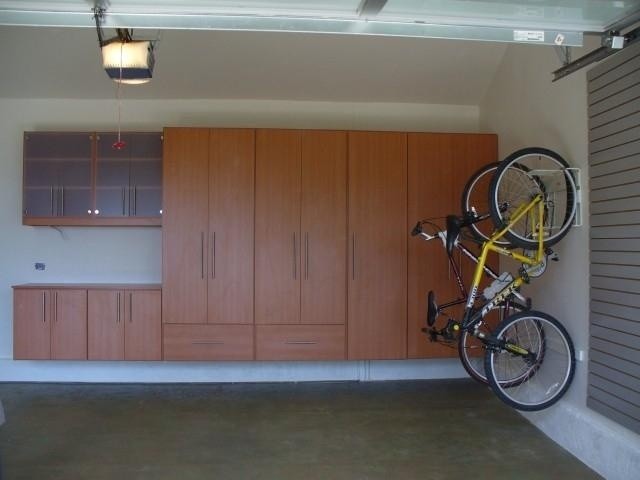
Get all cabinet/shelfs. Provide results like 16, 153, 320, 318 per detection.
406, 131, 498, 358
94, 132, 163, 226
87, 282, 162, 362
162, 128, 255, 362
254, 128, 348, 362
348, 129, 407, 362
13, 285, 88, 363
23, 132, 94, 225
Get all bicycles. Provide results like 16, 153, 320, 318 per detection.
414, 144, 578, 412
408, 159, 551, 388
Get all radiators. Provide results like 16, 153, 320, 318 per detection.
584, 41, 639, 437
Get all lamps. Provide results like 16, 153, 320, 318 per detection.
97, 29, 158, 87
